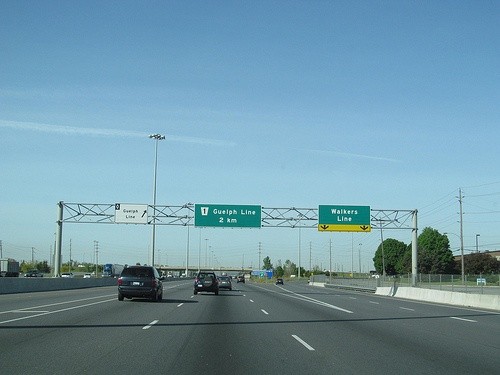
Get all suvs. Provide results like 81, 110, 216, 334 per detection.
193, 272, 219, 295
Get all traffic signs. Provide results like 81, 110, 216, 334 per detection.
115, 203, 148, 224
195, 205, 262, 228
318, 205, 371, 232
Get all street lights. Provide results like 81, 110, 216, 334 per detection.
149, 133, 166, 267
444, 231, 464, 285
184, 202, 194, 278
476, 233, 481, 253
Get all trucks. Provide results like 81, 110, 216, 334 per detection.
102, 264, 123, 278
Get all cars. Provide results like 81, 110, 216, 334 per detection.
83, 273, 92, 279
218, 276, 232, 290
61, 272, 74, 278
276, 278, 284, 285
160, 270, 184, 279
237, 275, 245, 284
117, 265, 166, 302
24, 269, 44, 278
